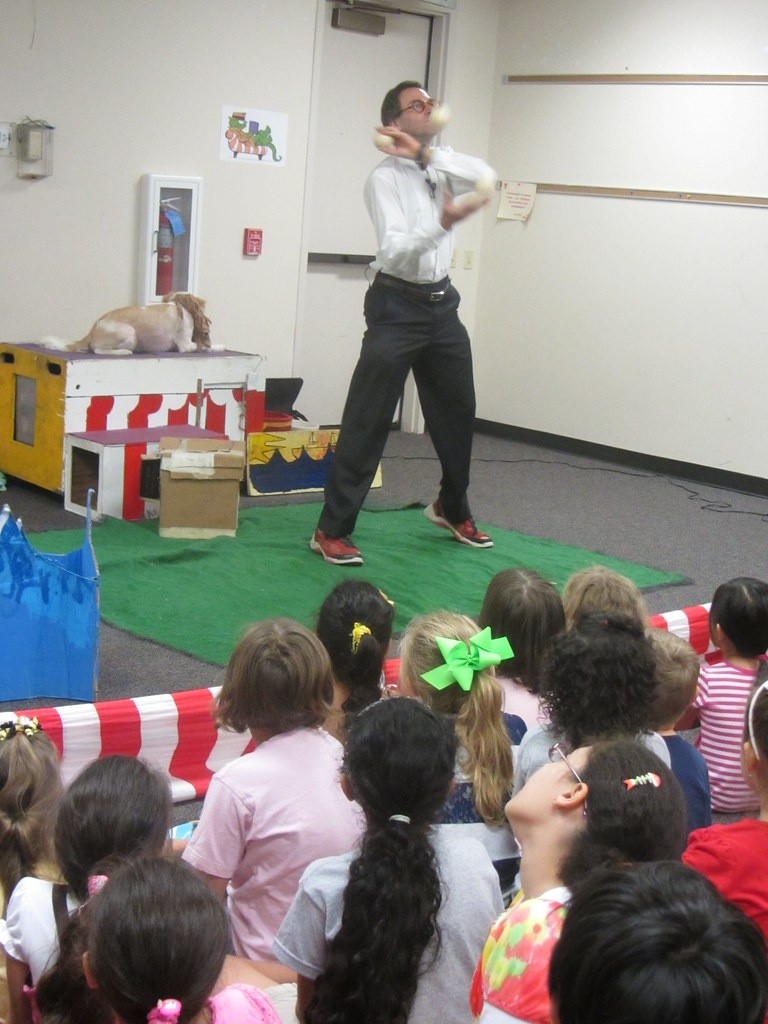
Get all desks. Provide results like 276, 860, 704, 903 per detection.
0, 343, 267, 494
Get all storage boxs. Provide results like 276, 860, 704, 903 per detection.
159, 435, 246, 540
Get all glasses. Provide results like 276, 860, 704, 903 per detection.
548, 741, 589, 819
400, 99, 441, 114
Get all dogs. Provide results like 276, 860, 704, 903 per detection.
40, 291, 233, 356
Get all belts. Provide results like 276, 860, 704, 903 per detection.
375, 274, 450, 303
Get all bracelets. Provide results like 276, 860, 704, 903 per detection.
416, 143, 426, 162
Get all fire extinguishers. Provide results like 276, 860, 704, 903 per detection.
156, 197, 181, 297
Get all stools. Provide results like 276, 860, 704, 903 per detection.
64, 424, 230, 522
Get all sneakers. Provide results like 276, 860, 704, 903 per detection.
310, 527, 363, 567
424, 497, 495, 548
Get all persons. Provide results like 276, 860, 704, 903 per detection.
0, 565, 768, 1024
546, 856, 767, 1023
308, 80, 496, 565
270, 695, 509, 1024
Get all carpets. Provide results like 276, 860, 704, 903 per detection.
24, 501, 695, 668
